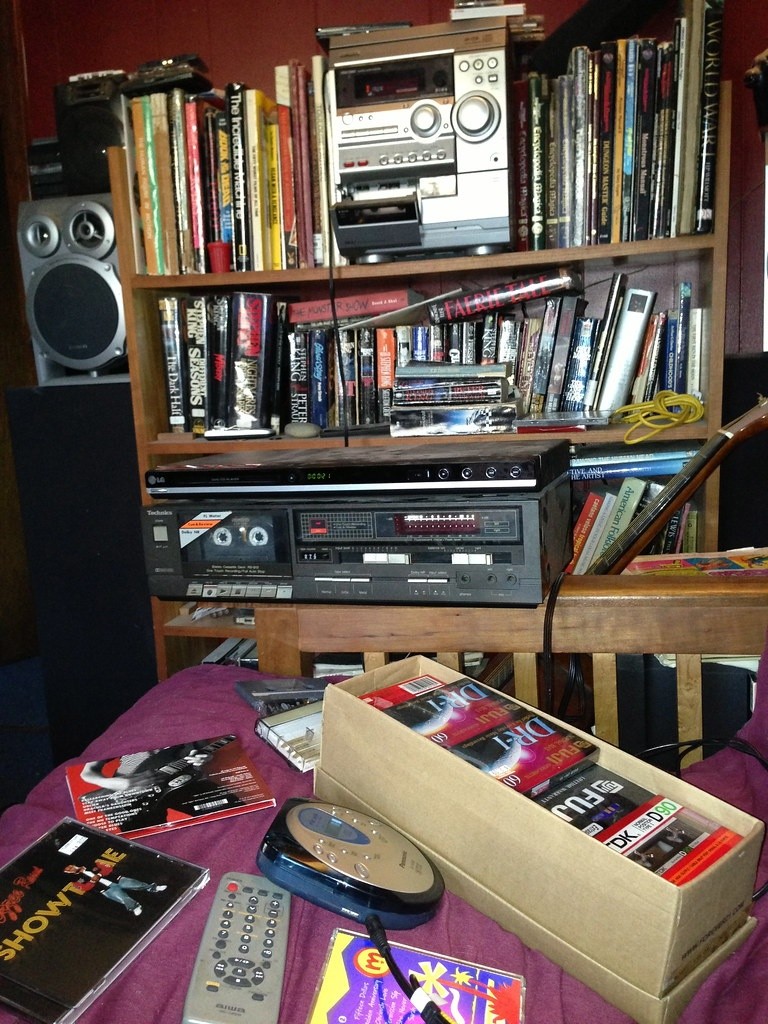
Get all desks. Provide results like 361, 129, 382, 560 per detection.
1, 665, 768, 1024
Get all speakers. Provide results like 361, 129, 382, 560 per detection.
16, 192, 132, 386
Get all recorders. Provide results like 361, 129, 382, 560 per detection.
146, 471, 578, 604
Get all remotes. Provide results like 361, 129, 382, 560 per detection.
182, 872, 291, 1024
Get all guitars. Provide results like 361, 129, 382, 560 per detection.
80, 735, 238, 833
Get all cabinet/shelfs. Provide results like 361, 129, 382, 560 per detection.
105, 81, 733, 684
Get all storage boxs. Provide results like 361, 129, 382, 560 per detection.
312, 654, 764, 1024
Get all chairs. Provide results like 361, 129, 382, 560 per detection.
254, 567, 767, 774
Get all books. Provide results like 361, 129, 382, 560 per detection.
121, 53, 333, 275
517, 2, 719, 253
157, 266, 710, 439
560, 398, 768, 578
203, 636, 365, 678
